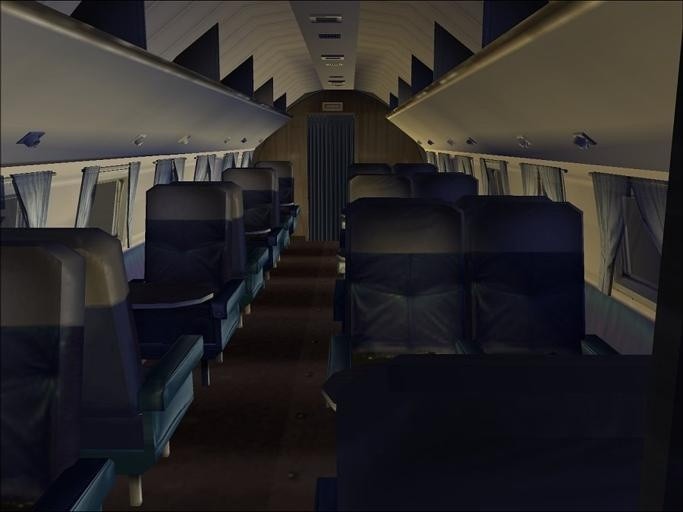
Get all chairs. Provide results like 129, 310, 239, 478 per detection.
0, 160, 301, 512
315, 162, 682, 512
